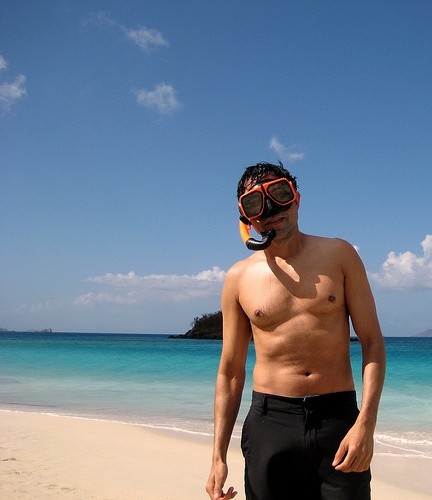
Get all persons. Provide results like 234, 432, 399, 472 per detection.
205, 160, 384, 500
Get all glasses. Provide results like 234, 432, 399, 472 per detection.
239, 177, 296, 224
